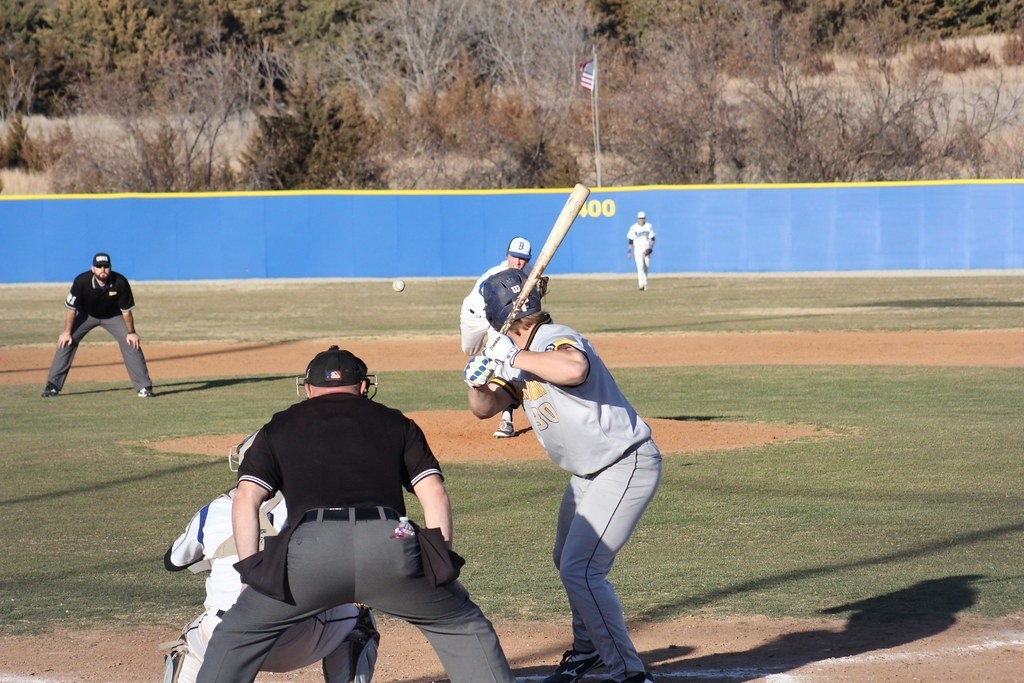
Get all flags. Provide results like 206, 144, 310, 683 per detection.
580, 62, 594, 92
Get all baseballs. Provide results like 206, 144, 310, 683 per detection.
393, 279, 405, 293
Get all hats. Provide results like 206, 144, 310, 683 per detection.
93, 253, 111, 266
304, 346, 368, 387
637, 211, 646, 219
508, 237, 533, 260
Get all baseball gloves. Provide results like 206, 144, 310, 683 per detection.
643, 248, 652, 256
535, 276, 549, 298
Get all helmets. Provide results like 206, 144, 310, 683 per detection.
484, 267, 541, 331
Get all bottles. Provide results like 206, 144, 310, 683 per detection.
394, 517, 415, 539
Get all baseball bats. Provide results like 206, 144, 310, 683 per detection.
500, 182, 591, 334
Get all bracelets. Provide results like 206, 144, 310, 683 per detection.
128, 331, 135, 334
628, 249, 631, 253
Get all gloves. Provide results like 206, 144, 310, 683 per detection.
463, 355, 496, 390
481, 325, 522, 367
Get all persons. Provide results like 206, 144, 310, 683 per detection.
460, 237, 549, 439
627, 211, 656, 291
464, 268, 663, 683
193, 345, 514, 683
41, 253, 153, 397
165, 430, 381, 683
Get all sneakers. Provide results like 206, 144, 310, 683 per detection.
598, 673, 653, 683
41, 385, 59, 397
137, 386, 153, 397
493, 419, 515, 438
542, 644, 605, 683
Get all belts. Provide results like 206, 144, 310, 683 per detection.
217, 609, 226, 618
300, 508, 400, 525
